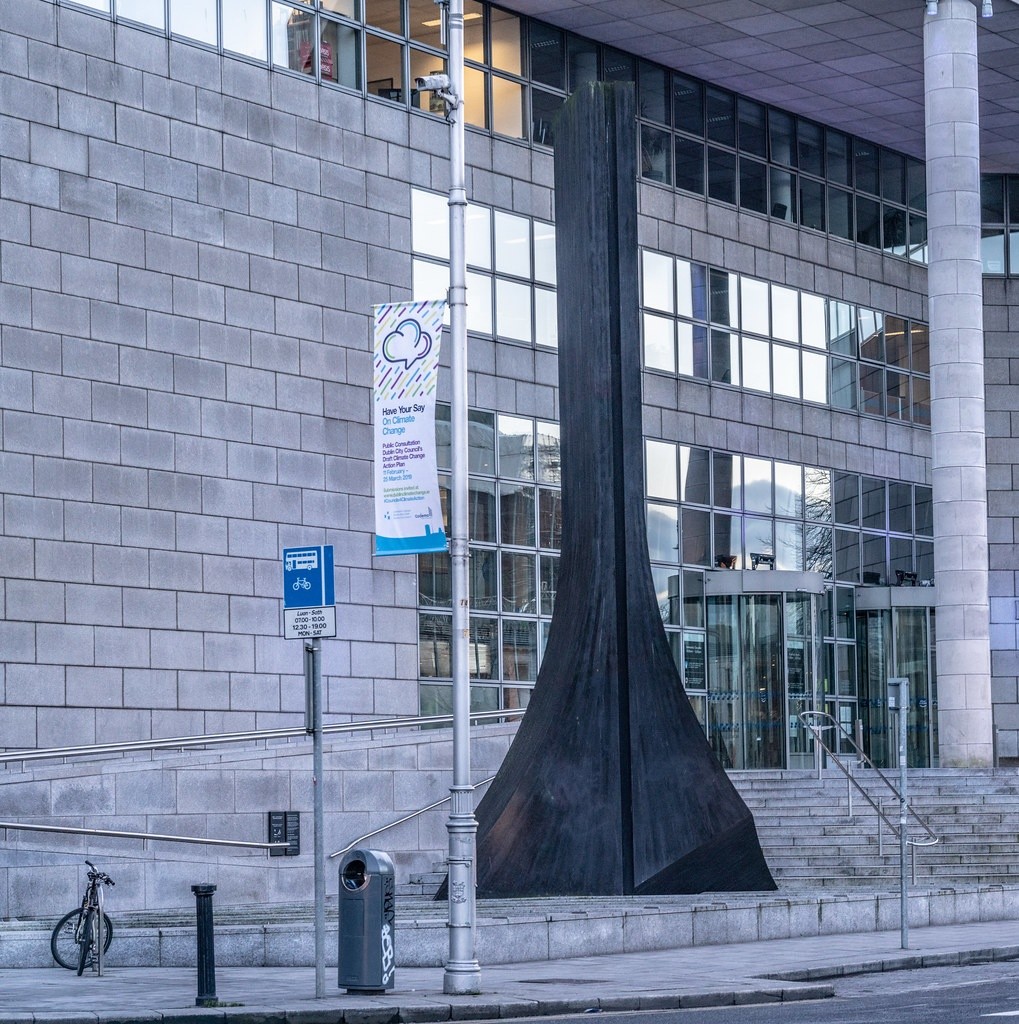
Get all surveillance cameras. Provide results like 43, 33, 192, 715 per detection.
414, 74, 451, 91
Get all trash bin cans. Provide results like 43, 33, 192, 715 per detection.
338, 849, 397, 994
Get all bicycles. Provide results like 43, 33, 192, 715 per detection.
51, 860, 115, 977
